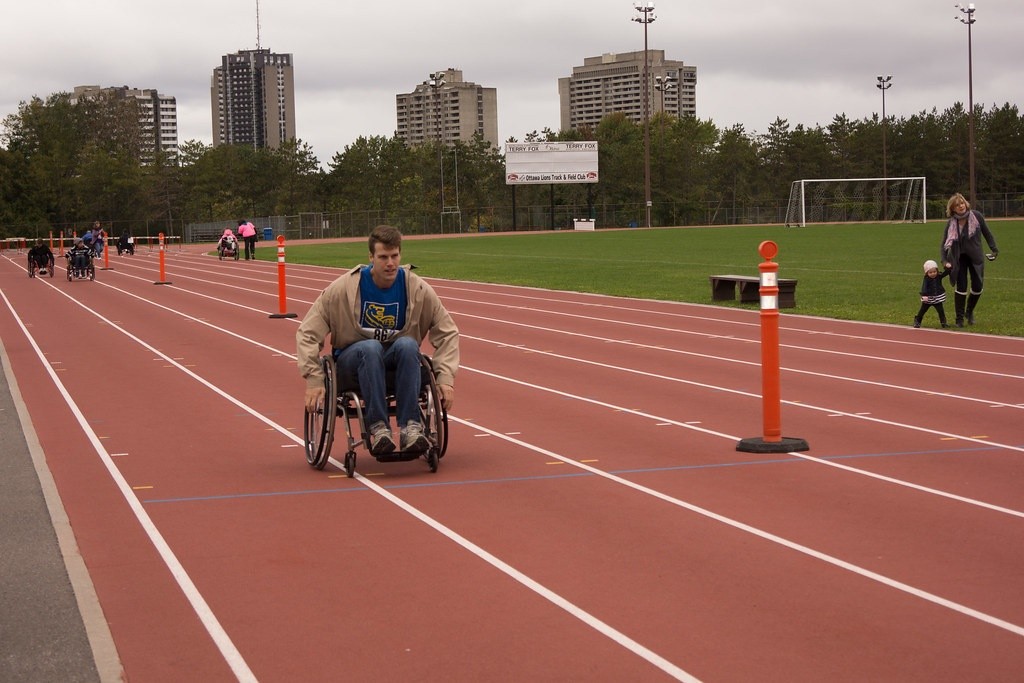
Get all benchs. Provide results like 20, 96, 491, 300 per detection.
709, 275, 798, 308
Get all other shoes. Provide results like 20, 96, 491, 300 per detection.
75, 272, 79, 277
39, 268, 47, 274
252, 254, 255, 260
94, 256, 101, 260
942, 322, 950, 328
914, 317, 921, 327
82, 272, 85, 277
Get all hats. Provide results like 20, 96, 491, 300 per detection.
924, 260, 938, 273
73, 237, 83, 246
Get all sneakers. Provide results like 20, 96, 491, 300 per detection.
400, 419, 429, 454
368, 420, 396, 454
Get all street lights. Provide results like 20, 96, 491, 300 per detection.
876, 75, 893, 221
953, 2, 976, 211
631, 0, 658, 228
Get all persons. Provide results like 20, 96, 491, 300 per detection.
295, 225, 460, 455
64, 220, 112, 281
117, 227, 134, 252
28, 237, 54, 275
217, 219, 258, 260
913, 193, 1000, 328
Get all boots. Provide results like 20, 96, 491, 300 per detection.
965, 288, 983, 324
955, 290, 967, 327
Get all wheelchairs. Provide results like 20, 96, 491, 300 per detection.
218, 241, 240, 261
303, 352, 448, 478
67, 251, 96, 282
117, 241, 136, 257
28, 254, 55, 279
